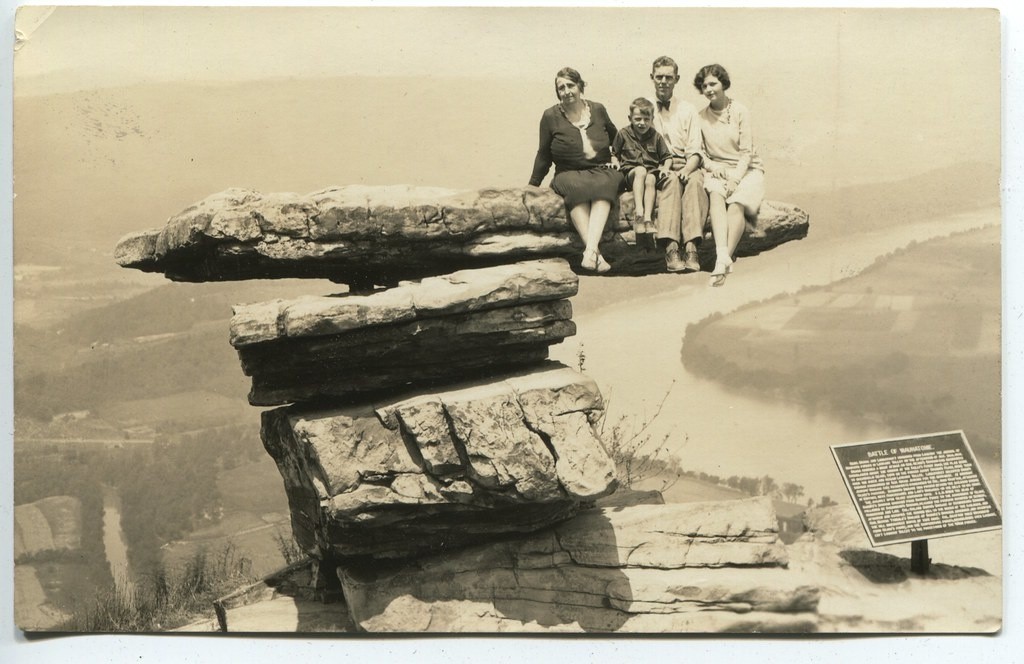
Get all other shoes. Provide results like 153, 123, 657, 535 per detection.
665, 249, 685, 271
643, 220, 657, 234
709, 254, 732, 286
596, 254, 611, 273
633, 214, 645, 234
682, 250, 701, 273
581, 249, 597, 270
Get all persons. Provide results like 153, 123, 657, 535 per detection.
528, 56, 765, 277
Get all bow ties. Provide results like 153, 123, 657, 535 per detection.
657, 101, 670, 112
